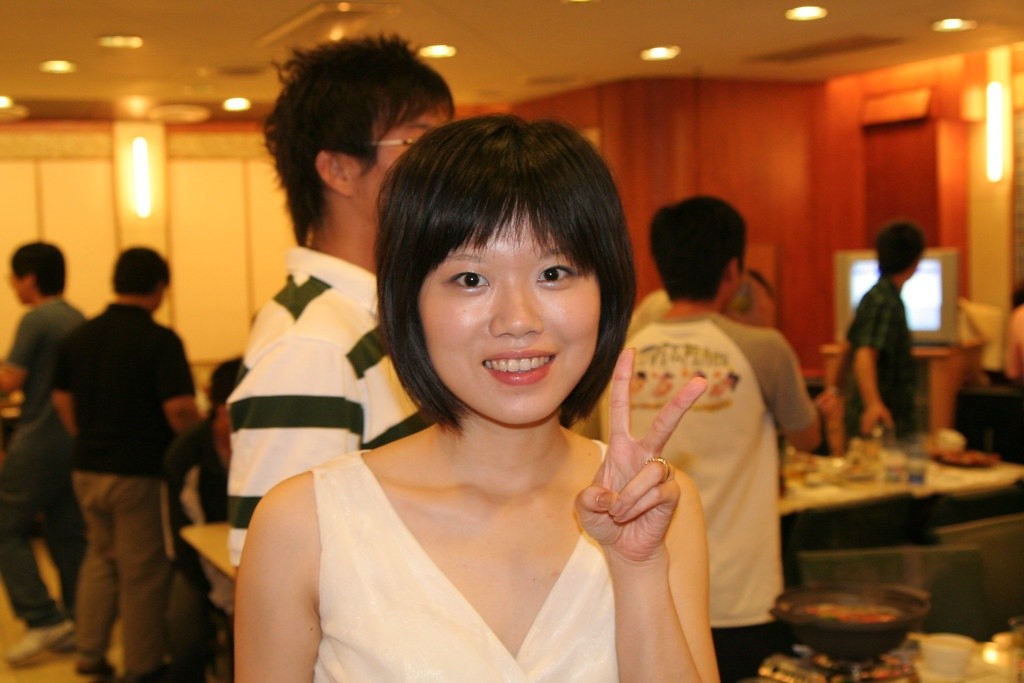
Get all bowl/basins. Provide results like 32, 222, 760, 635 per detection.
769, 582, 933, 657
920, 629, 976, 683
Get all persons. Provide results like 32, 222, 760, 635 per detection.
169, 353, 243, 525
226, 38, 456, 568
233, 112, 720, 683
47, 247, 199, 683
598, 194, 821, 683
840, 222, 926, 438
0, 241, 86, 662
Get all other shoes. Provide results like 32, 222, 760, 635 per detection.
2, 618, 76, 664
136, 664, 171, 682
75, 651, 116, 676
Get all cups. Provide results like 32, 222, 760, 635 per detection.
847, 437, 879, 481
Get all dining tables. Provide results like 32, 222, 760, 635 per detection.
778, 443, 1024, 587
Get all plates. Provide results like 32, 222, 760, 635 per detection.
936, 447, 1001, 467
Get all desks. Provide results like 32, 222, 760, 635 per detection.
819, 338, 987, 436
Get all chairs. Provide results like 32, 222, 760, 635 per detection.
795, 512, 1023, 643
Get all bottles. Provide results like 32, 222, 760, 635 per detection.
872, 420, 927, 485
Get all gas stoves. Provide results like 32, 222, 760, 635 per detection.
758, 644, 918, 682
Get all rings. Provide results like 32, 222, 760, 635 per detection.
645, 457, 670, 483
